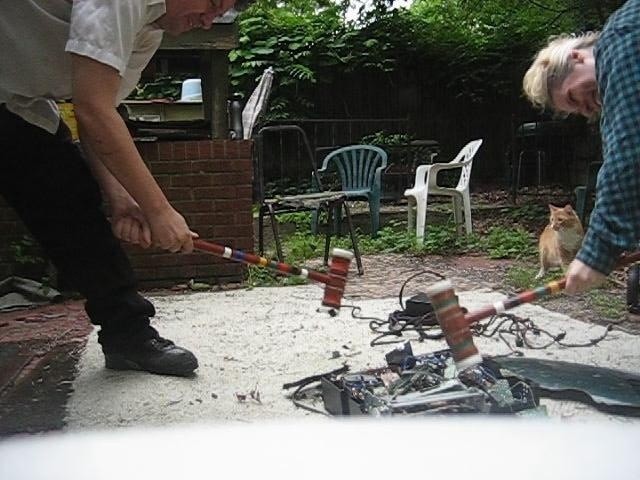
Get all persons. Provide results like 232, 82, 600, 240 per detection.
0, 0, 244, 378
521, 0, 639, 299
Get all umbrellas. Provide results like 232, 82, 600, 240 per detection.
242, 64, 276, 141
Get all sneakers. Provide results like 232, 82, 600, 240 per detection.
104, 325, 201, 377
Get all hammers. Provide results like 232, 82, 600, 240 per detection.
105, 216, 354, 310
425, 246, 640, 370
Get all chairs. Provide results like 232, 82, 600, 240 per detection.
255, 122, 483, 275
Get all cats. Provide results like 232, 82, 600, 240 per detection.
534, 204, 585, 282
